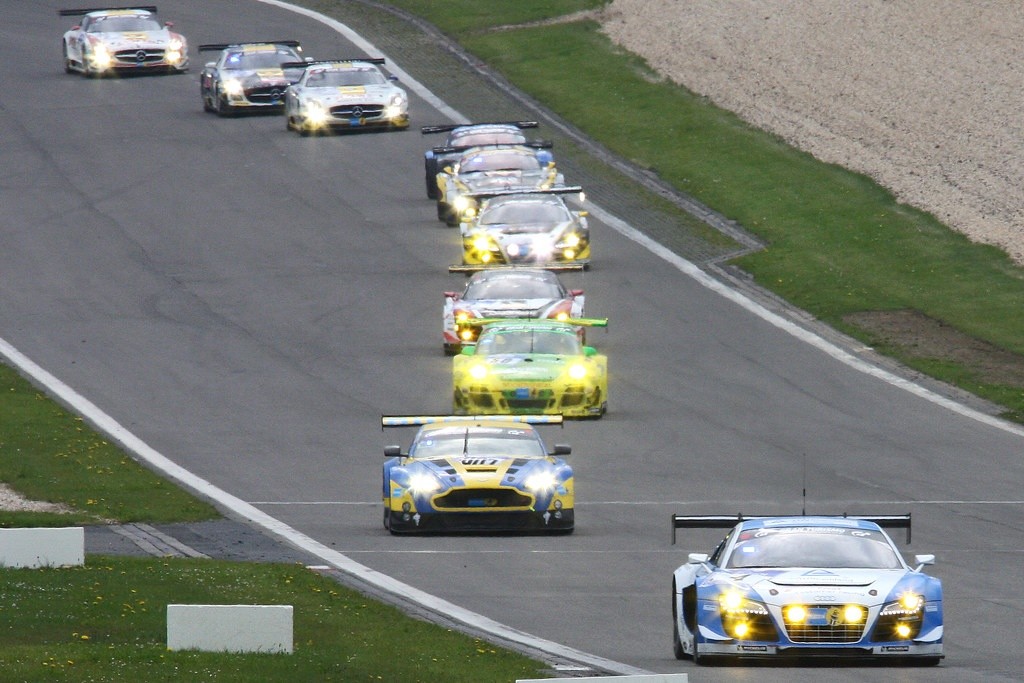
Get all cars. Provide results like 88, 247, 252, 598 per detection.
458, 184, 591, 278
442, 263, 586, 352
59, 4, 190, 78
380, 415, 575, 537
281, 56, 410, 137
672, 512, 945, 667
421, 119, 539, 201
196, 39, 315, 116
453, 316, 610, 419
431, 141, 566, 227
